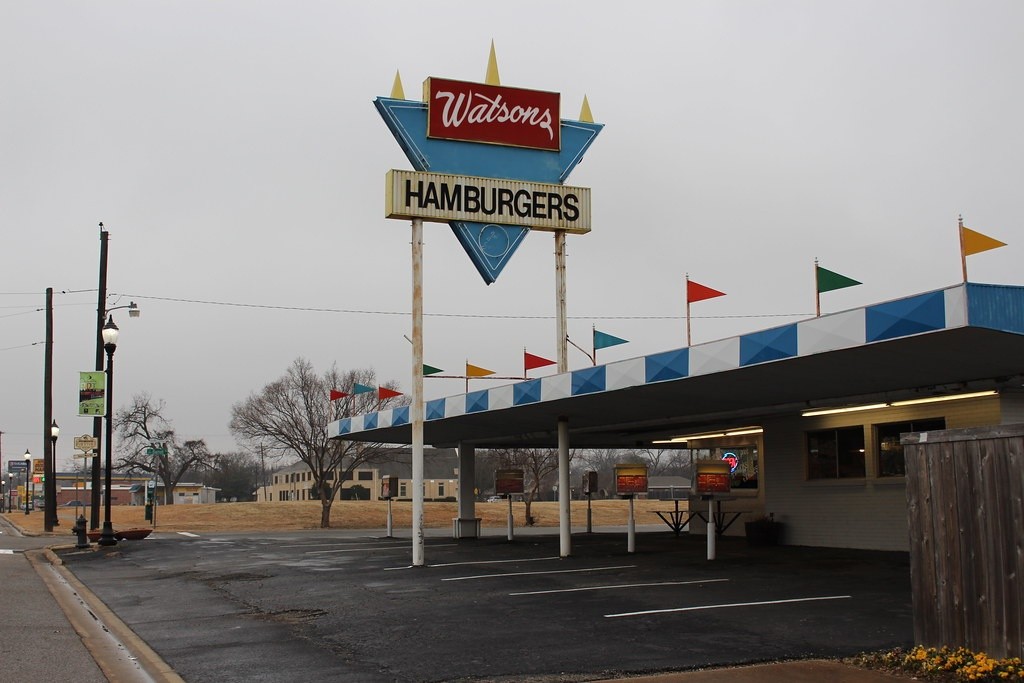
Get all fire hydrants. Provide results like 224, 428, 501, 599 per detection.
71, 514, 90, 548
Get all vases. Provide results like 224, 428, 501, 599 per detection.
745, 521, 780, 545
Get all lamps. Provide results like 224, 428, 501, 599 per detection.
651, 427, 766, 444
801, 401, 891, 417
892, 390, 1002, 406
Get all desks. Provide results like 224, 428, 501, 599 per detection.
649, 509, 751, 540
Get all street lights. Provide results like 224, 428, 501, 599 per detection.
51, 419, 60, 526
24, 449, 32, 516
1, 478, 6, 513
90, 303, 142, 498
8, 471, 14, 513
96, 313, 120, 547
